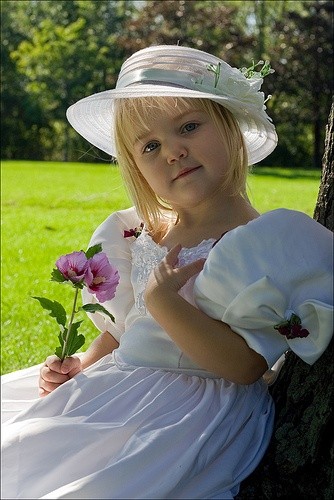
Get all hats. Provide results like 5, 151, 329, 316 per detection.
66, 45, 278, 166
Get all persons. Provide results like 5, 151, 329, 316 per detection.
0, 46, 334, 500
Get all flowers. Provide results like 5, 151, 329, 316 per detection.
192, 58, 275, 122
30, 242, 120, 357
